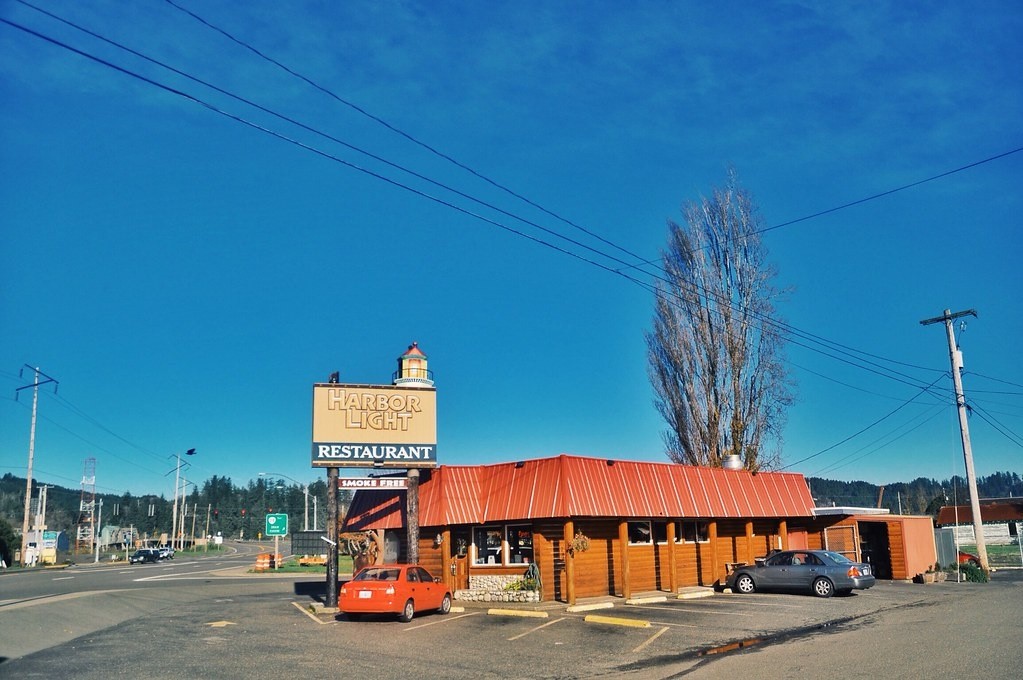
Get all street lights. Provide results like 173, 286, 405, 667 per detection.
276, 485, 318, 531
258, 472, 309, 531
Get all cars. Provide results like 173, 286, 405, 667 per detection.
956, 548, 980, 569
725, 551, 875, 598
337, 563, 453, 622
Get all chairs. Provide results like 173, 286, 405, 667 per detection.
795, 558, 802, 565
385, 576, 397, 580
408, 574, 413, 580
805, 557, 811, 563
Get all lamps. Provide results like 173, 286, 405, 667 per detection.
434, 533, 443, 544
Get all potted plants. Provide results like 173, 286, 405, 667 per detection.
923, 562, 946, 584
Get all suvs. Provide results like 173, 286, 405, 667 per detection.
158, 547, 175, 560
128, 549, 160, 565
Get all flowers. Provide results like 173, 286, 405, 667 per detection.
568, 533, 590, 552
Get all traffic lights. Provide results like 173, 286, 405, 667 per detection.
268, 508, 273, 513
241, 508, 246, 518
214, 509, 219, 521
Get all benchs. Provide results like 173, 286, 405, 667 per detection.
299, 558, 325, 566
